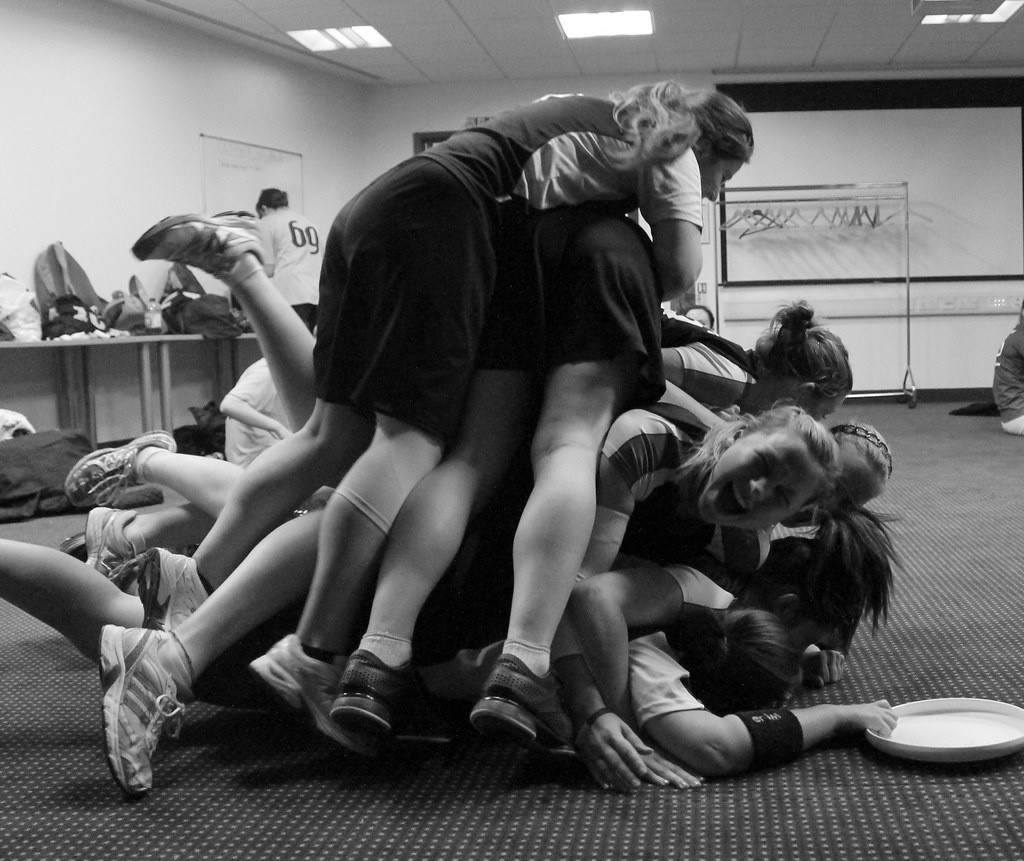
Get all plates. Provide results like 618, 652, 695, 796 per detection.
865, 698, 1024, 762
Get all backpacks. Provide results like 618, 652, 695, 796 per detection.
0, 240, 245, 342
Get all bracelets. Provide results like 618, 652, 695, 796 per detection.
575, 706, 614, 747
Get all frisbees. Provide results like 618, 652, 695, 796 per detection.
858, 698, 1024, 763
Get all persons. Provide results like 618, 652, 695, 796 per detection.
1, 80, 896, 806
993, 299, 1024, 435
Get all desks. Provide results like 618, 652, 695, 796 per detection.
0, 332, 262, 439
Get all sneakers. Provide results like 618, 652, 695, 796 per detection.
138, 548, 210, 633
470, 655, 577, 756
333, 650, 456, 742
64, 430, 177, 506
59, 531, 88, 562
99, 624, 196, 794
249, 633, 368, 755
120, 567, 138, 597
133, 211, 275, 276
84, 507, 145, 591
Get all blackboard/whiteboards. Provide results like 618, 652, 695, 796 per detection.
708, 105, 1024, 289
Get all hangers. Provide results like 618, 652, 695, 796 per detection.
719, 196, 933, 238
